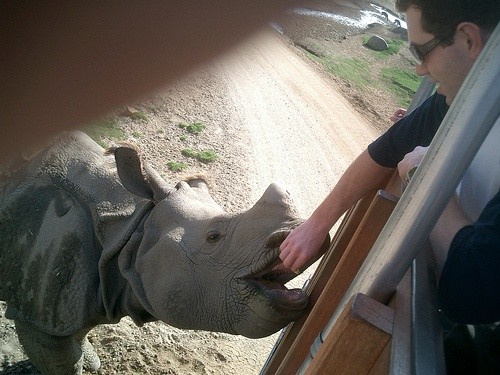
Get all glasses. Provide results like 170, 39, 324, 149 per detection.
408, 34, 442, 65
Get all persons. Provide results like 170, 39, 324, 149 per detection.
277, 1, 500, 324
389, 108, 407, 123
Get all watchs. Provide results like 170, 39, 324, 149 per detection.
406, 163, 419, 185
